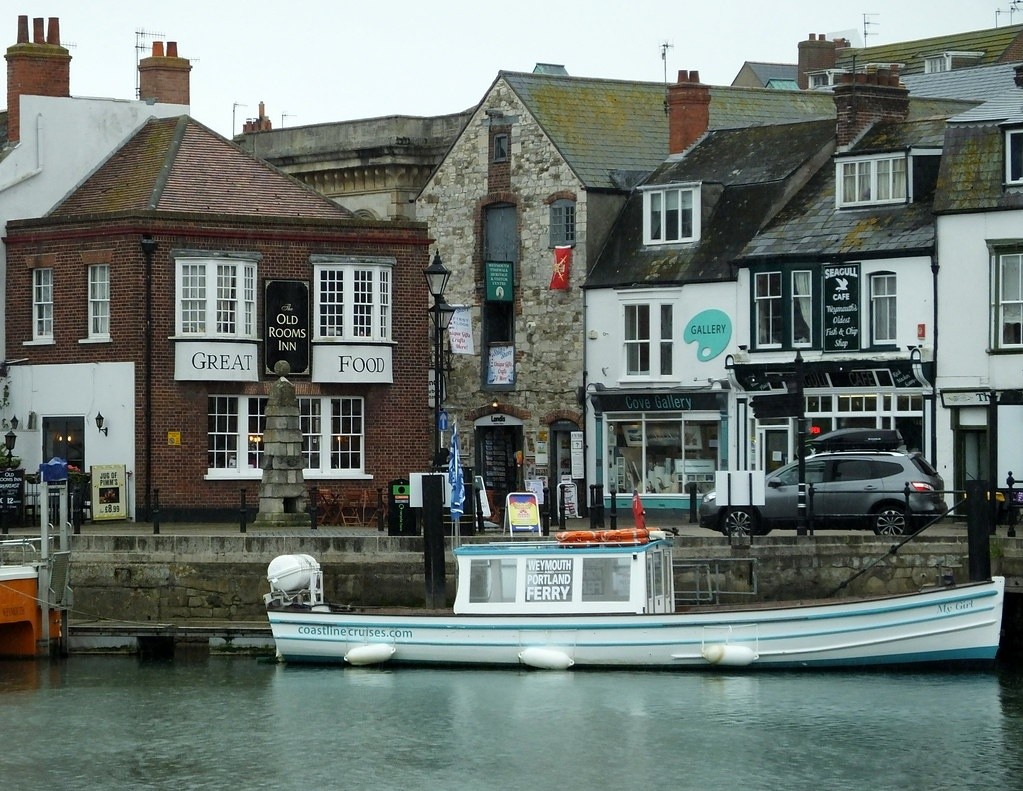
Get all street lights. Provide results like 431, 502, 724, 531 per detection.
423, 252, 457, 470
747, 374, 808, 538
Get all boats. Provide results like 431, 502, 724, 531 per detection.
0, 536, 62, 657
261, 525, 1007, 674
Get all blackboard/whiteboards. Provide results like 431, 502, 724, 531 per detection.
473, 476, 490, 517
525, 479, 545, 504
409, 472, 454, 508
0, 469, 25, 514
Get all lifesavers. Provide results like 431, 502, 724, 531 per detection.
556, 531, 605, 548
604, 527, 660, 547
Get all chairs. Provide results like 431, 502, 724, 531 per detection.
486, 490, 501, 524
318, 489, 389, 528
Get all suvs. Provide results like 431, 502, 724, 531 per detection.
699, 426, 947, 537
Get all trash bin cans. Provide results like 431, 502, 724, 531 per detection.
388, 479, 421, 536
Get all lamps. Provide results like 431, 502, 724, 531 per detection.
10, 414, 19, 429
491, 397, 498, 407
95, 410, 108, 436
907, 346, 917, 350
738, 345, 747, 350
